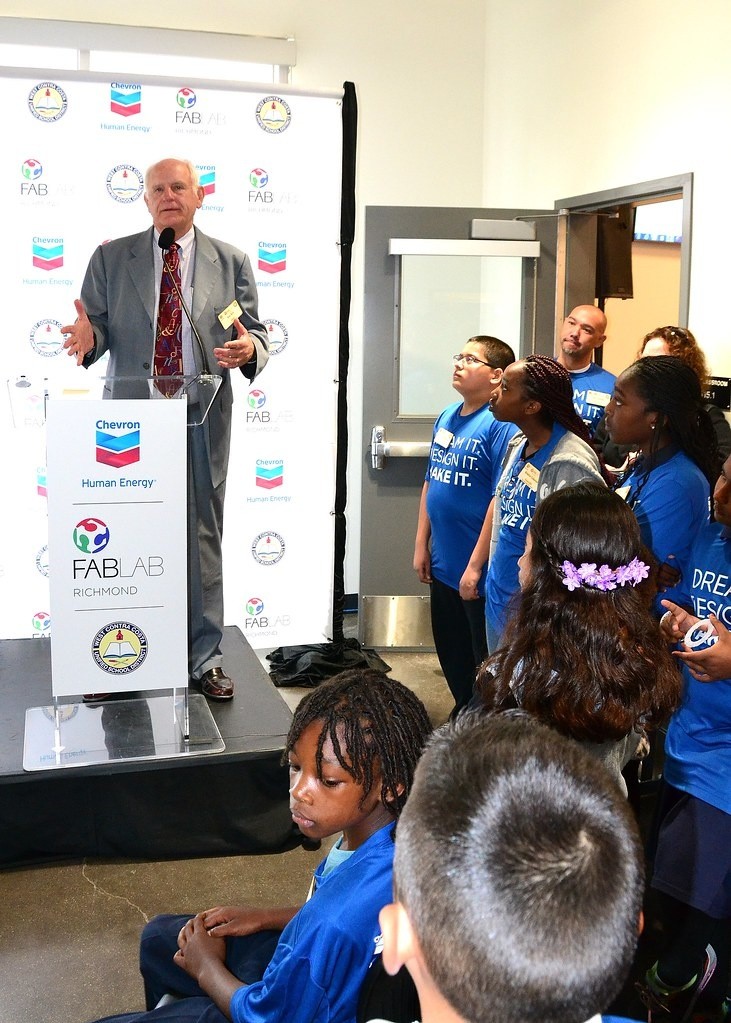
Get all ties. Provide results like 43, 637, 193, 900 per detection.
153, 244, 183, 400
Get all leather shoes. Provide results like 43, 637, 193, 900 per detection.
83, 691, 107, 701
190, 668, 234, 701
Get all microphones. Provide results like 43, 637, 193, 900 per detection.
157, 228, 207, 376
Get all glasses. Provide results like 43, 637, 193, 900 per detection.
453, 354, 498, 369
667, 327, 687, 343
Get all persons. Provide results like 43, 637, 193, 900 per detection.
61, 157, 271, 702
378, 702, 646, 1023
413, 304, 731, 1023
93, 666, 436, 1023
